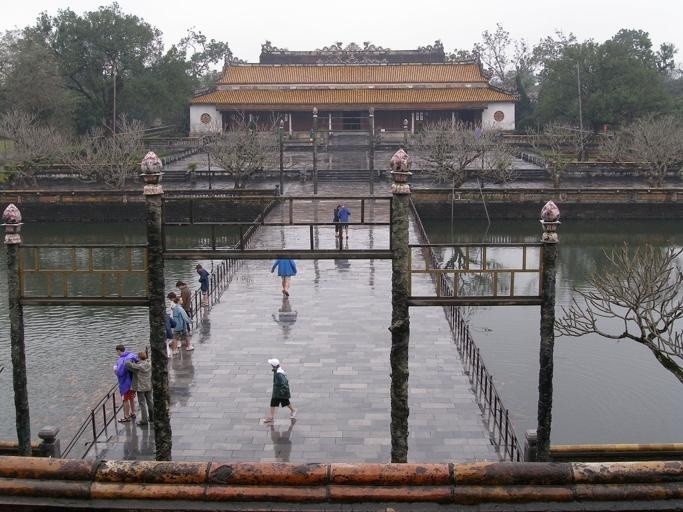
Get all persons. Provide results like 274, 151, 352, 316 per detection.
111, 345, 137, 423
332, 205, 341, 238
197, 305, 211, 343
120, 417, 138, 460
164, 280, 195, 359
337, 203, 351, 239
167, 345, 195, 406
126, 351, 154, 425
265, 416, 297, 463
271, 295, 297, 339
270, 247, 296, 297
333, 236, 350, 271
262, 358, 298, 422
137, 422, 158, 462
195, 263, 210, 308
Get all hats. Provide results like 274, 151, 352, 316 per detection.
267, 359, 279, 367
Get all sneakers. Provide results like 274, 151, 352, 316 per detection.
147, 416, 153, 425
262, 417, 274, 423
168, 343, 194, 359
288, 409, 296, 419
136, 421, 148, 425
282, 290, 289, 296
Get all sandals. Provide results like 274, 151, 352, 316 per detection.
117, 417, 130, 422
129, 413, 136, 418
